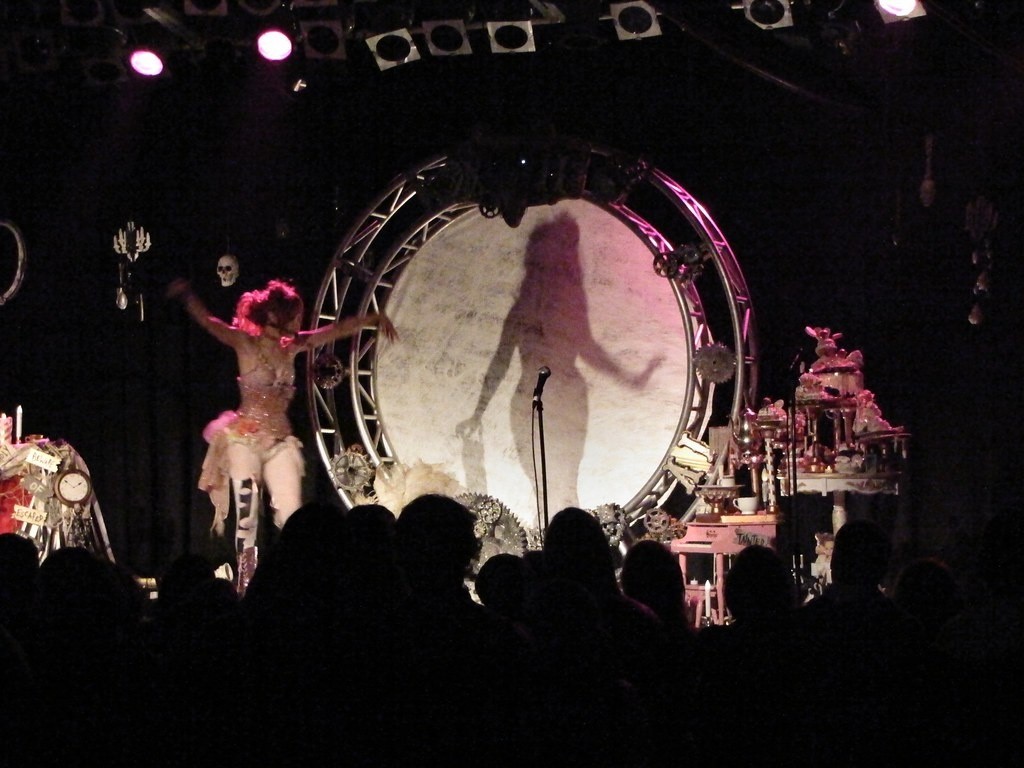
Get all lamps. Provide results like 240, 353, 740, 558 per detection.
0, 0, 928, 94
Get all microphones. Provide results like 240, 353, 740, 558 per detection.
532, 366, 551, 409
795, 347, 805, 361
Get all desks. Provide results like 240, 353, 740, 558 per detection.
777, 472, 900, 586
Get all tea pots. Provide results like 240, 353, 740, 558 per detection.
726, 408, 762, 452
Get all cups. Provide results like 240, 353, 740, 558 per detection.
717, 479, 734, 487
733, 497, 757, 514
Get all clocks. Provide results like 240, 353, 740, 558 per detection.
54, 469, 93, 507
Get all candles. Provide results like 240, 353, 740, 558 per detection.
690, 578, 698, 585
705, 580, 711, 617
16, 405, 22, 437
0, 413, 12, 448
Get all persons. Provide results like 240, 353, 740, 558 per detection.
168, 275, 402, 598
0, 416, 50, 481
0, 494, 1024, 768
